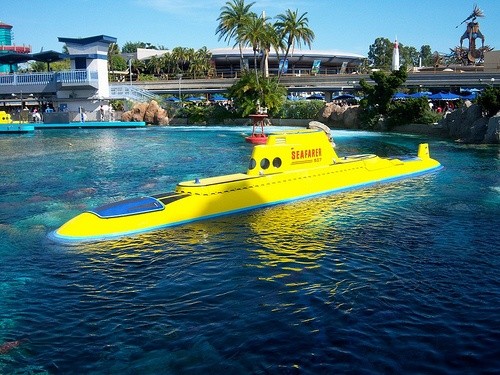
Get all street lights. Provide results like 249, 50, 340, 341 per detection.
176, 74, 183, 106
127, 55, 134, 86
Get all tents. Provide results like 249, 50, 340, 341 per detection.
394, 91, 427, 98
428, 91, 460, 105
163, 97, 181, 102
332, 93, 355, 105
209, 96, 228, 101
184, 96, 201, 100
462, 92, 480, 101
306, 93, 326, 100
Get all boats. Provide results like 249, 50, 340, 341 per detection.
56, 128, 441, 238
0, 111, 28, 124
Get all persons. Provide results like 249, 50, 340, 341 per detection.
33, 107, 37, 121
8, 107, 21, 121
437, 101, 459, 115
428, 101, 434, 109
42, 101, 56, 122
216, 100, 231, 107
100, 105, 105, 122
